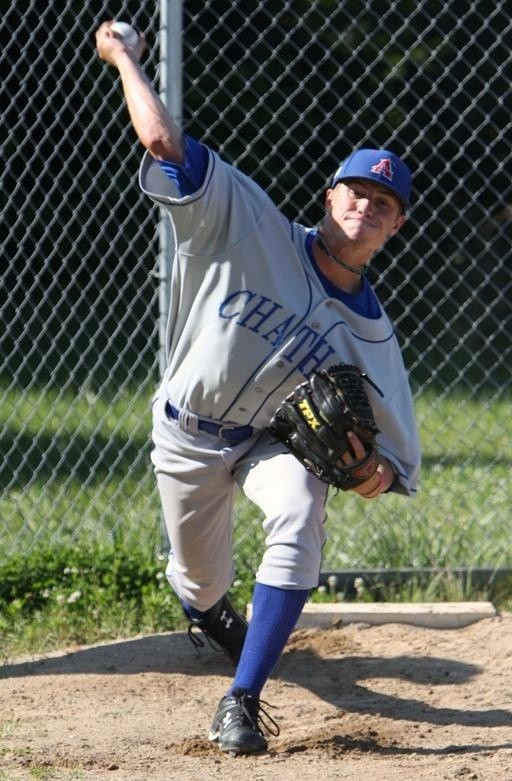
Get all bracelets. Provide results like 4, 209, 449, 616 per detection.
351, 470, 384, 499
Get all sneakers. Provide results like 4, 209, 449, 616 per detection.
206, 694, 268, 755
177, 594, 248, 667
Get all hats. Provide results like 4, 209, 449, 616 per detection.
329, 147, 412, 218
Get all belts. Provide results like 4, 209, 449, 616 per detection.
148, 398, 254, 443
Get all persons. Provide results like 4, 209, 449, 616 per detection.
96, 23, 420, 756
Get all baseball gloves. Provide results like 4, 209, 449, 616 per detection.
261, 362, 386, 492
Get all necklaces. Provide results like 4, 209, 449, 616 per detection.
311, 230, 369, 287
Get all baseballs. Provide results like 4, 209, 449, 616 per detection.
109, 22, 138, 49
